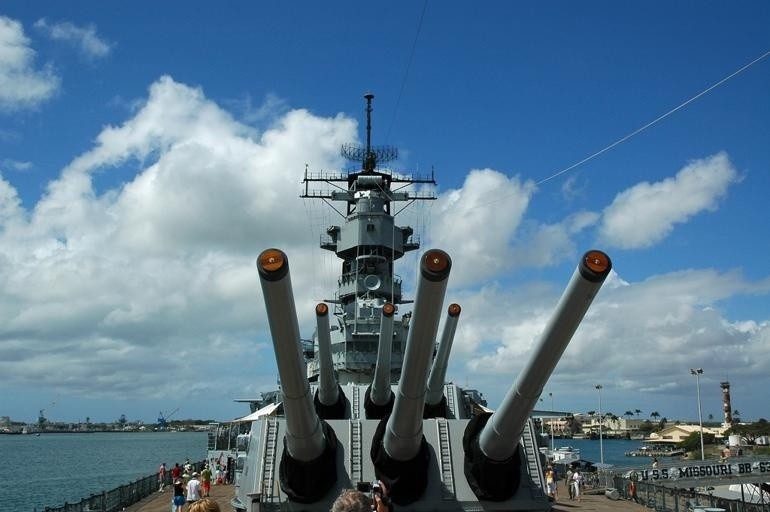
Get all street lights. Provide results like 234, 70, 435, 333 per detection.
595, 384, 604, 464
548, 392, 554, 450
690, 367, 704, 460
540, 398, 544, 433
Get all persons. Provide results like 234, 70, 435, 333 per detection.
543, 464, 582, 504
719, 451, 731, 463
324, 479, 390, 511
156, 451, 235, 512
650, 457, 662, 466
734, 444, 743, 455
723, 444, 731, 456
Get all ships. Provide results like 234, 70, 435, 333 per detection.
32, 91, 770, 511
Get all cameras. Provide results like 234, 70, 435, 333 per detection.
357, 480, 381, 511
